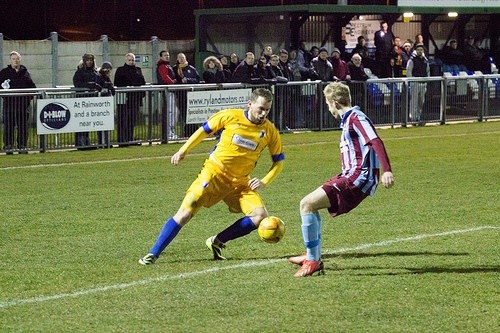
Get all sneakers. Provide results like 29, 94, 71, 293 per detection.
137, 253, 157, 265
205, 235, 227, 260
289, 255, 324, 277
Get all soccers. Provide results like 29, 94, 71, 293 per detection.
258, 217, 285, 243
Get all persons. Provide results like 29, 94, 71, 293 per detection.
156, 50, 179, 140
223, 52, 242, 80
233, 52, 258, 88
72, 53, 104, 150
203, 56, 225, 91
310, 48, 334, 131
374, 20, 395, 78
296, 42, 319, 80
346, 53, 369, 114
278, 47, 301, 134
351, 35, 369, 68
386, 35, 428, 78
258, 55, 288, 134
406, 45, 430, 126
172, 52, 199, 137
444, 40, 467, 71
137, 88, 285, 267
97, 61, 116, 149
260, 45, 274, 64
114, 53, 146, 148
464, 35, 493, 74
288, 81, 394, 278
329, 47, 348, 81
0, 50, 38, 154
217, 55, 229, 79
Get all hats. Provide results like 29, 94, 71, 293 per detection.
402, 40, 412, 47
318, 46, 328, 52
101, 61, 112, 68
415, 42, 424, 47
331, 47, 341, 54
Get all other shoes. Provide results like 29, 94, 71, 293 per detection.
6, 146, 28, 154
78, 140, 141, 150
